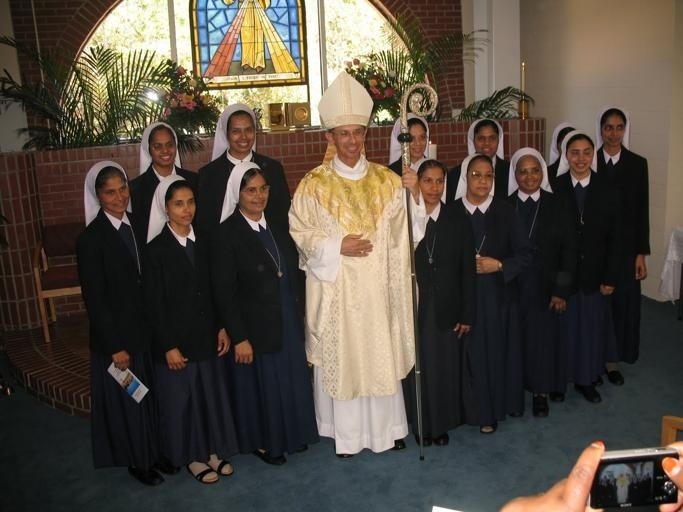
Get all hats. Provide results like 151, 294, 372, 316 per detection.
316, 69, 376, 132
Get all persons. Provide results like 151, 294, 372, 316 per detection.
209, 160, 317, 466
452, 150, 533, 435
494, 438, 682, 512
74, 160, 181, 487
550, 128, 617, 405
286, 114, 420, 460
402, 155, 477, 448
448, 115, 511, 196
589, 104, 650, 389
125, 121, 195, 229
595, 468, 655, 506
381, 110, 433, 182
195, 101, 291, 225
542, 121, 578, 181
505, 146, 573, 421
142, 172, 235, 484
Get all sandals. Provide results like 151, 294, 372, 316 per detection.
205, 457, 236, 477
183, 459, 219, 485
481, 423, 498, 435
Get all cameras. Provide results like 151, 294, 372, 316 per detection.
585, 449, 680, 508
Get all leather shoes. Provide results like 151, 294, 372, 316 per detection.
603, 365, 624, 386
574, 383, 602, 404
531, 389, 550, 418
389, 439, 407, 450
548, 390, 567, 403
252, 447, 286, 465
414, 430, 433, 448
127, 465, 164, 487
433, 433, 450, 447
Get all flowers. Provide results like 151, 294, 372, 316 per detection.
343, 58, 401, 124
142, 59, 222, 155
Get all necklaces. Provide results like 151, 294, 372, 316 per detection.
578, 207, 583, 226
424, 232, 436, 265
529, 201, 539, 238
129, 226, 140, 275
474, 235, 486, 258
261, 222, 283, 279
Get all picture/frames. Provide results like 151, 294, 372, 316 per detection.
188, 0, 307, 90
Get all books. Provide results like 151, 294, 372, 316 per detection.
105, 360, 150, 405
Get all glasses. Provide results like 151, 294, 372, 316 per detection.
471, 169, 495, 182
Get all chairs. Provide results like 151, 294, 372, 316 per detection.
32, 239, 84, 343
